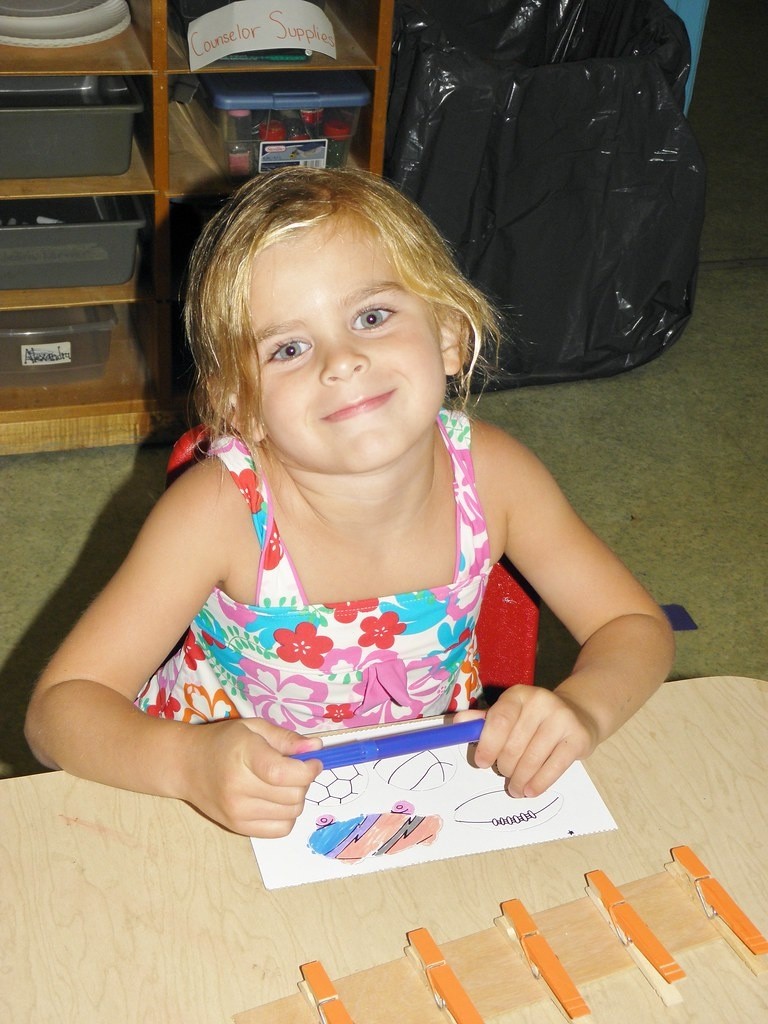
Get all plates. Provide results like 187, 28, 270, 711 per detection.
0, 0, 132, 49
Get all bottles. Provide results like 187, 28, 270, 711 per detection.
225, 108, 351, 179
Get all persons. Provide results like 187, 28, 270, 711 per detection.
18, 160, 679, 840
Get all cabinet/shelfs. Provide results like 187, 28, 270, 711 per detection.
0, 0, 395, 459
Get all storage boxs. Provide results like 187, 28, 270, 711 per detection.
0, 75, 148, 383
185, 71, 374, 189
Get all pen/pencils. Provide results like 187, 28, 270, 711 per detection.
289, 717, 483, 772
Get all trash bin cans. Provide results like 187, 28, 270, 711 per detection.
391, 0, 692, 393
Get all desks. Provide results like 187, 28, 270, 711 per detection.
0, 676, 768, 1021
